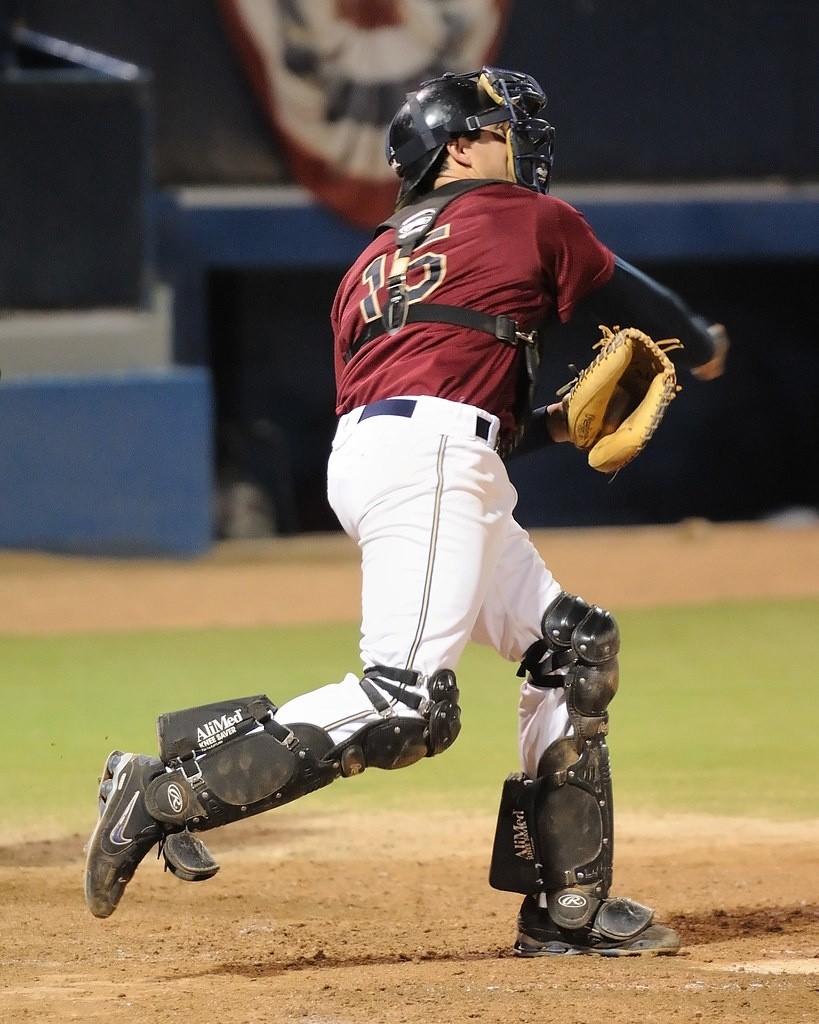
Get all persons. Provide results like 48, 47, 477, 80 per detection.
84, 68, 726, 957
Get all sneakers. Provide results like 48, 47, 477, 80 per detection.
512, 888, 680, 958
84, 750, 171, 919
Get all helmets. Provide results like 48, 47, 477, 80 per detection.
385, 78, 527, 210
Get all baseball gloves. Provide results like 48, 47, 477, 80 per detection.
564, 327, 677, 473
